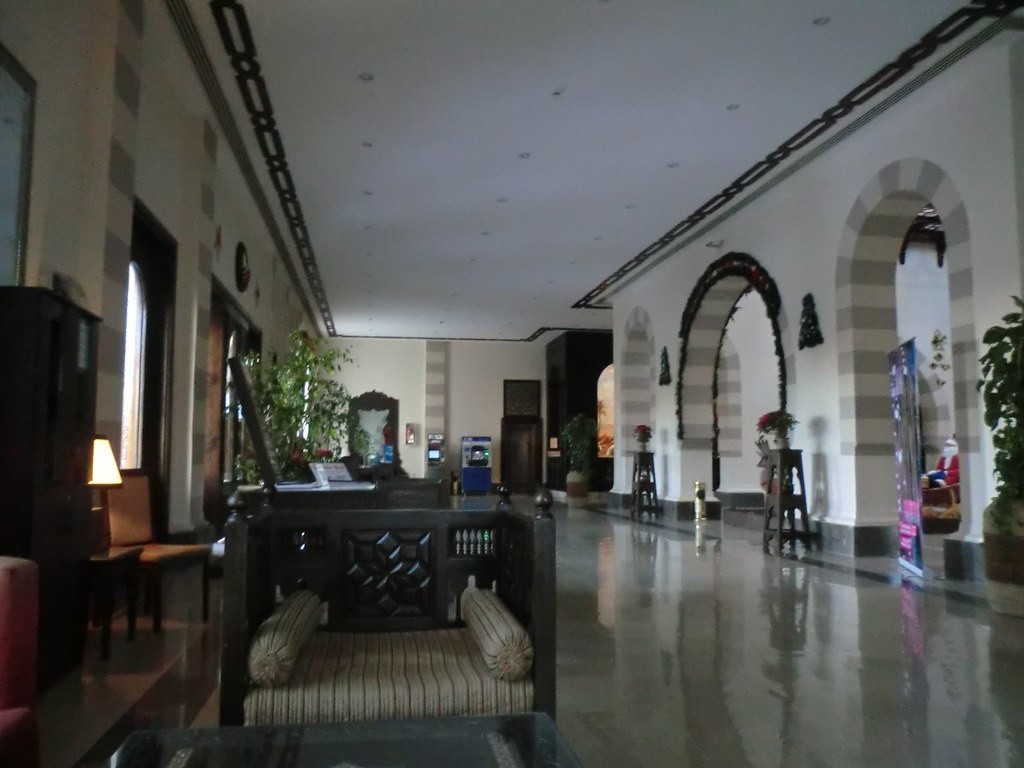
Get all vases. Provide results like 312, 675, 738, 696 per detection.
767, 435, 790, 451
637, 442, 648, 452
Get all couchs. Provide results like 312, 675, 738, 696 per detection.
0, 556, 45, 768
288, 462, 448, 511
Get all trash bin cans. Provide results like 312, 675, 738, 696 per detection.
694, 481, 706, 519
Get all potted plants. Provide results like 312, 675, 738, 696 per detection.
560, 410, 600, 507
972, 295, 1024, 616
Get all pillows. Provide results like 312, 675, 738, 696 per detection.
248, 588, 323, 685
462, 586, 535, 679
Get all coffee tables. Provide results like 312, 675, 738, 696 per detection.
131, 544, 207, 633
98, 711, 587, 768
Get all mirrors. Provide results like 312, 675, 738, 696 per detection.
347, 390, 398, 464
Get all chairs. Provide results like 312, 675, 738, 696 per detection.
218, 501, 556, 759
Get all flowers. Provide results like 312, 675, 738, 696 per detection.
757, 411, 796, 437
632, 424, 652, 442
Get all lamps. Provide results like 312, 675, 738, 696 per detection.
88, 437, 121, 549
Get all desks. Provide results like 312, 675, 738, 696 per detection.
84, 544, 143, 662
631, 451, 658, 523
764, 449, 811, 551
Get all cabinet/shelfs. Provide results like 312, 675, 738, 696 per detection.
0, 284, 105, 725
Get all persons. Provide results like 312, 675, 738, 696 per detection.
928, 438, 960, 488
